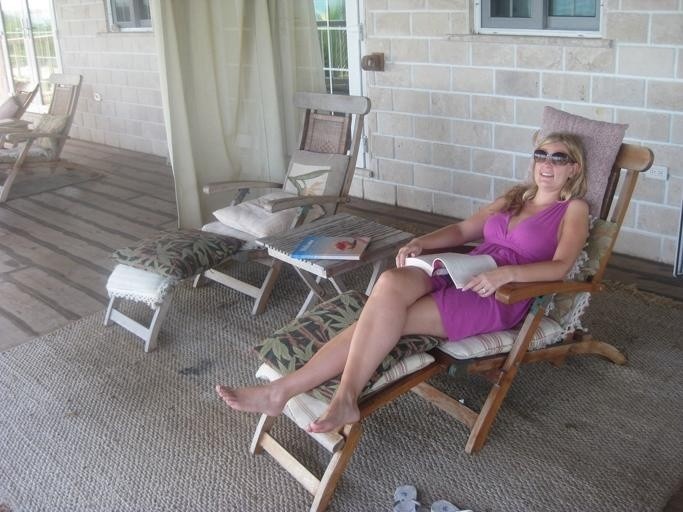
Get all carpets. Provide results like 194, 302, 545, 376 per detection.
0, 221, 682, 512
0, 158, 102, 201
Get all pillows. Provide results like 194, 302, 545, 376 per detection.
248, 288, 439, 403
110, 226, 247, 278
522, 105, 628, 219
213, 191, 326, 239
437, 312, 565, 361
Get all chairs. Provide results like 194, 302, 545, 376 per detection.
246, 129, 655, 512
105, 90, 371, 353
0, 73, 82, 203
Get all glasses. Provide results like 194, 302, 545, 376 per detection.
533, 148, 576, 167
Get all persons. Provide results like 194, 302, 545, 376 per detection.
215, 131, 589, 434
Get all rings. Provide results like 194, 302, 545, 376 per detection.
481, 288, 485, 294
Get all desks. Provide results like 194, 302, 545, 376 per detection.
254, 213, 415, 322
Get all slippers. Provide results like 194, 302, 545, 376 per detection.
430, 497, 473, 511
391, 481, 419, 511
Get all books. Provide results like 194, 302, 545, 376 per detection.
404, 251, 499, 290
290, 235, 372, 260
